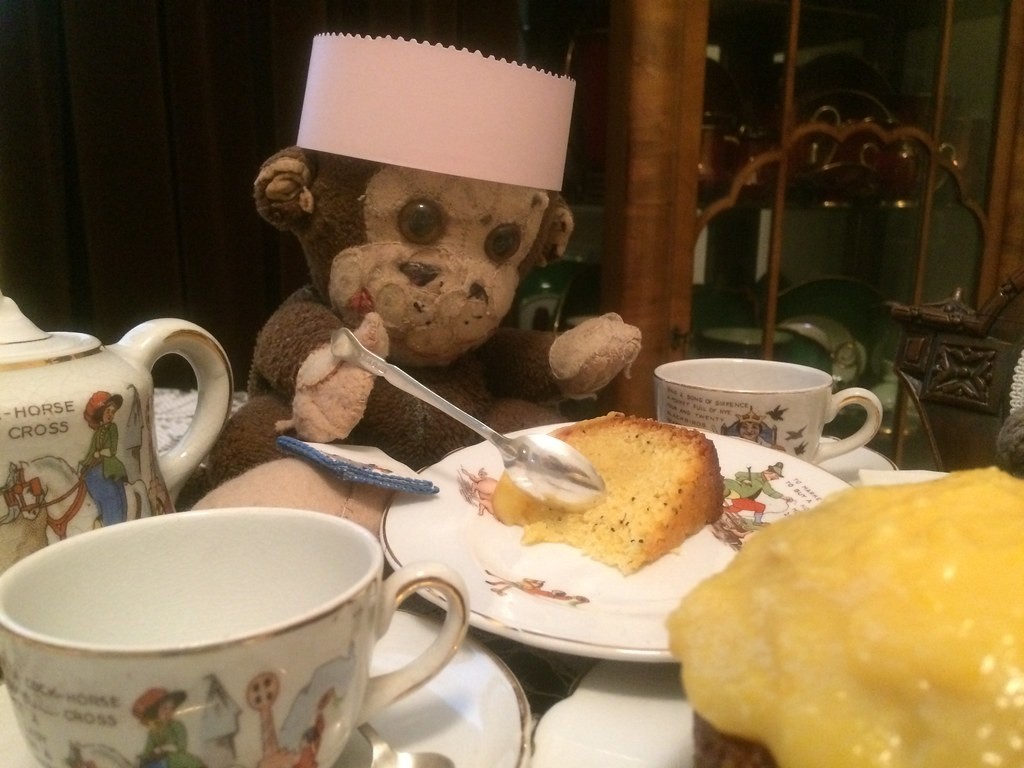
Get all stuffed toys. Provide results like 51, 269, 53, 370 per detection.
175, 147, 642, 537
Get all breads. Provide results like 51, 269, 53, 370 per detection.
494, 411, 725, 576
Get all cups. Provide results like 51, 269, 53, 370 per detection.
653, 358, 884, 468
1, 503, 481, 768
0, 286, 233, 596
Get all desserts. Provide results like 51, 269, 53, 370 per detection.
666, 469, 1024, 768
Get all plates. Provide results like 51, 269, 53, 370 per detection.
377, 415, 866, 662
523, 655, 704, 767
808, 434, 901, 490
3, 599, 531, 768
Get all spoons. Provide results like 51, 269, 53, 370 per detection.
356, 721, 457, 768
329, 329, 604, 511
